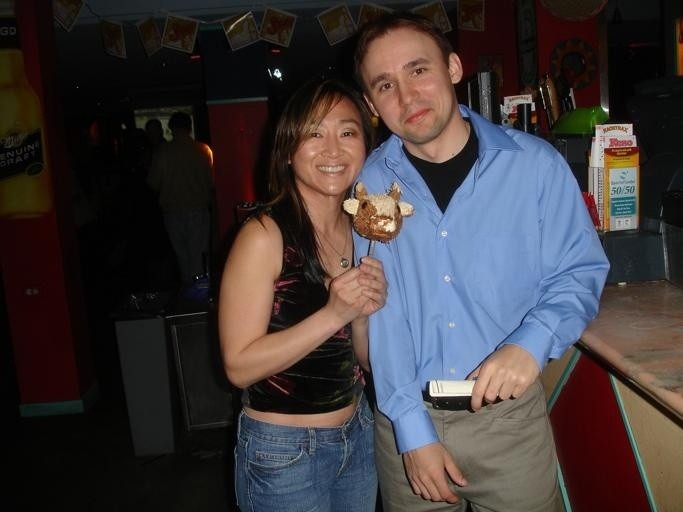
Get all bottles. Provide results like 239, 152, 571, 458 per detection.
0, 10, 53, 221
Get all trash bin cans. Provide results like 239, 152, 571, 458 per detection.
109, 288, 185, 458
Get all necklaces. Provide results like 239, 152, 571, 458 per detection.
316, 222, 351, 269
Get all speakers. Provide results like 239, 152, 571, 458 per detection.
172, 320, 234, 432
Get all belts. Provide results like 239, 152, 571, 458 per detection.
422, 393, 513, 411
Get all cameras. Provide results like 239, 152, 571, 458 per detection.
428, 380, 477, 410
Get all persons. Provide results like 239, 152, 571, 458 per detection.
93, 109, 219, 306
217, 76, 388, 512
349, 9, 610, 512
